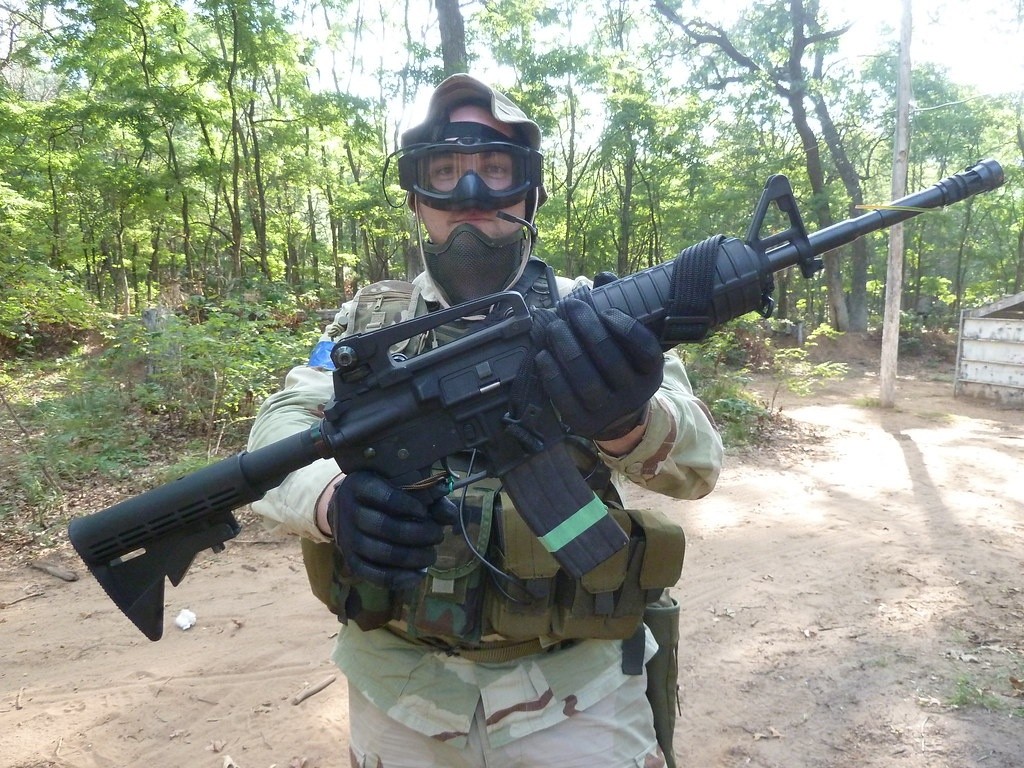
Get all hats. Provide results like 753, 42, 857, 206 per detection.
401, 72, 543, 152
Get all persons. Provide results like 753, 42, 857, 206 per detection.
240, 72, 723, 768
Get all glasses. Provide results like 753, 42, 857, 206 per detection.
390, 138, 545, 207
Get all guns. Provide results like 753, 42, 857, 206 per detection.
63, 159, 1009, 642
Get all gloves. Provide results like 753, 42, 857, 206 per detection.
533, 299, 667, 442
326, 469, 446, 593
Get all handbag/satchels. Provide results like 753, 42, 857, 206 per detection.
300, 476, 502, 652
480, 489, 687, 643
